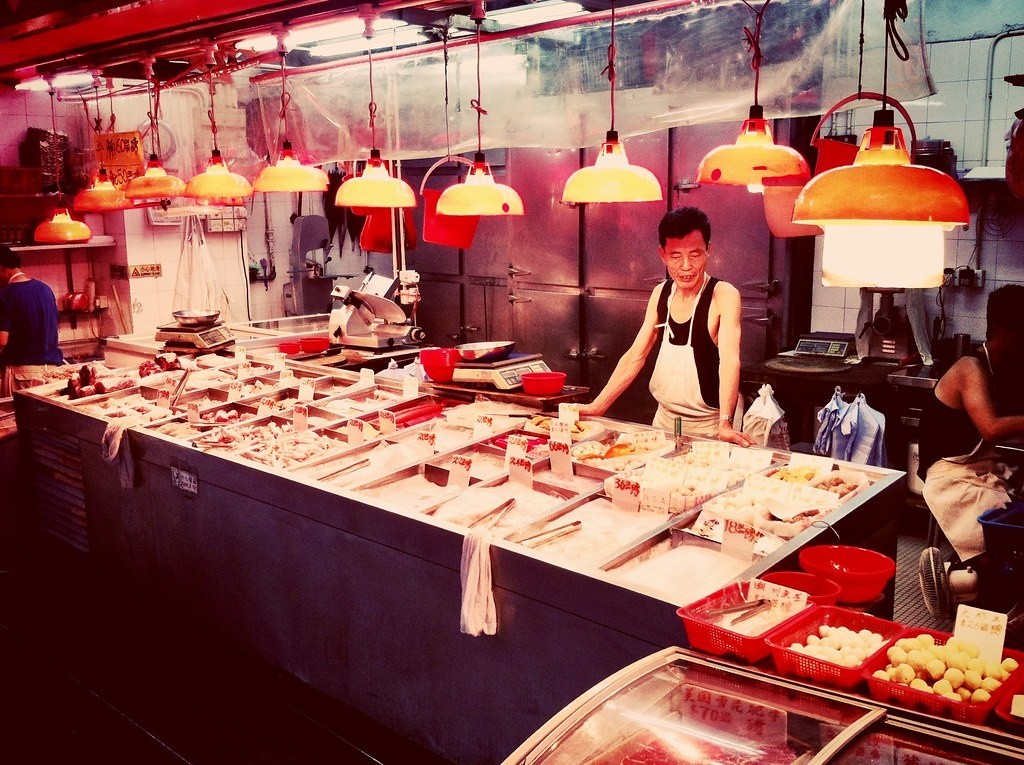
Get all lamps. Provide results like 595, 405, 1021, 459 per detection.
182, 45, 254, 199
792, 0, 971, 288
693, 0, 811, 193
124, 58, 186, 199
436, 0, 524, 215
561, 0, 664, 202
34, 76, 92, 243
72, 69, 131, 211
335, 5, 417, 207
253, 24, 329, 192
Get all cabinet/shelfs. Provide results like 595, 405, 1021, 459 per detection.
404, 115, 815, 425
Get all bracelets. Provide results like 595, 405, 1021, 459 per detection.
719, 415, 733, 423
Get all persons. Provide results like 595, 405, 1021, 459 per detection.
0, 246, 63, 397
578, 207, 758, 448
917, 284, 1024, 571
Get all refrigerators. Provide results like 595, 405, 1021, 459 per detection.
498, 648, 1021, 765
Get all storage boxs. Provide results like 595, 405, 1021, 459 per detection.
977, 500, 1024, 614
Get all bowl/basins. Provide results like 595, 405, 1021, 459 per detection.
420, 350, 458, 383
454, 342, 515, 361
759, 572, 841, 607
278, 343, 300, 354
521, 372, 566, 395
173, 310, 220, 325
799, 545, 895, 602
299, 337, 330, 353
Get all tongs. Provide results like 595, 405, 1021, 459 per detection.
516, 520, 582, 549
704, 599, 771, 626
467, 497, 516, 531
191, 441, 232, 449
190, 422, 227, 427
170, 369, 191, 406
317, 458, 371, 482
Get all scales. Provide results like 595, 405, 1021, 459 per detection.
155, 309, 236, 348
452, 341, 552, 390
794, 332, 856, 358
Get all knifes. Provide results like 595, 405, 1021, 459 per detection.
485, 413, 532, 418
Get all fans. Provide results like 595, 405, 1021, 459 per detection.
918, 547, 983, 621
